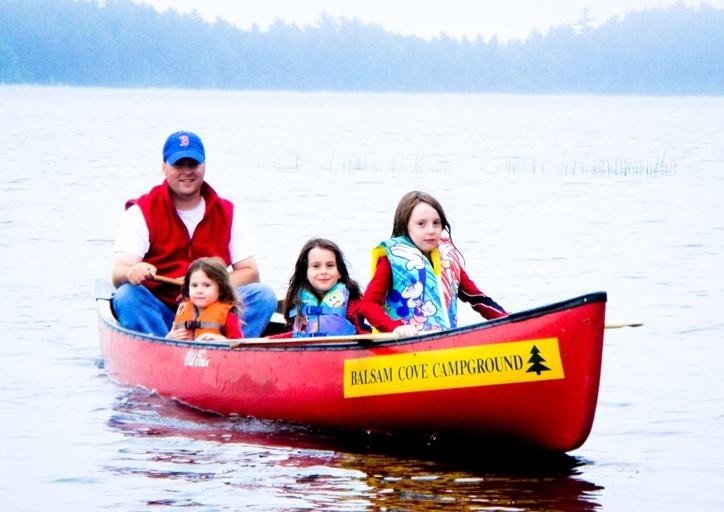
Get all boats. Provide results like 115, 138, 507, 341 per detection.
96, 290, 608, 453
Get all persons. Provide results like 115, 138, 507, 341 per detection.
111, 130, 278, 338
283, 238, 374, 339
359, 192, 510, 339
164, 258, 244, 343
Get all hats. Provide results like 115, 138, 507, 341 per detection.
163, 131, 204, 166
